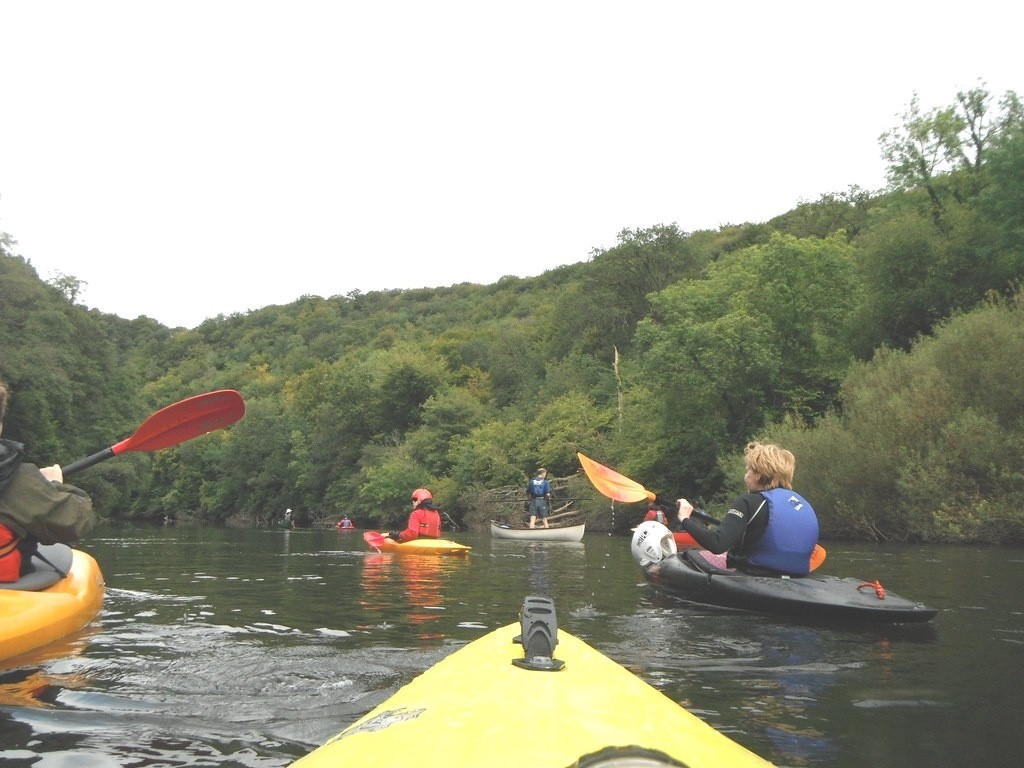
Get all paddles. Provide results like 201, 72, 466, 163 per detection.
58, 390, 245, 478
364, 531, 391, 548
578, 452, 826, 573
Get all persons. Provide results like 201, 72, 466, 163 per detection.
338, 514, 352, 527
0, 387, 96, 583
526, 468, 550, 529
285, 509, 295, 527
390, 489, 441, 544
676, 443, 819, 579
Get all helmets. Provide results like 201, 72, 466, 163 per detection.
631, 521, 678, 565
412, 489, 433, 503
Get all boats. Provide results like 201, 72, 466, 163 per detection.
378, 533, 472, 556
0, 542, 105, 664
335, 526, 354, 529
489, 519, 585, 541
672, 531, 699, 546
630, 526, 940, 625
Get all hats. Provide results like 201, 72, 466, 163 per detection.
286, 509, 292, 513
